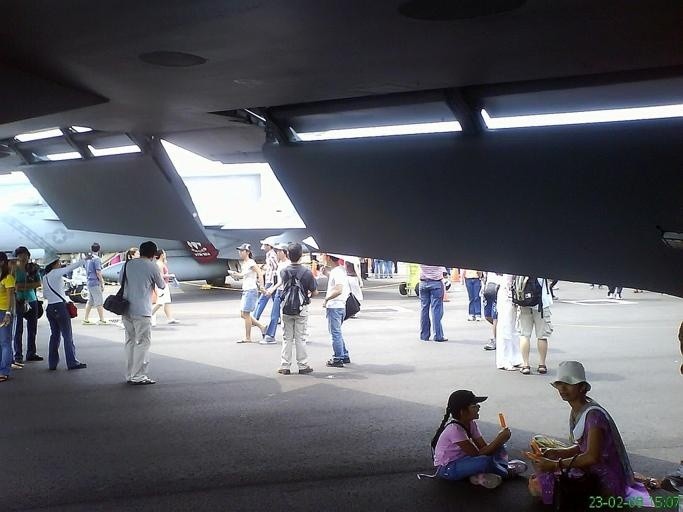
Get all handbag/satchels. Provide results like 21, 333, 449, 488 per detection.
67, 302, 77, 318
24, 301, 43, 321
552, 454, 602, 512
103, 295, 130, 315
343, 293, 360, 320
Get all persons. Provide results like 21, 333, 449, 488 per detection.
42, 250, 94, 371
1, 246, 44, 382
229, 240, 398, 376
118, 240, 180, 384
81, 242, 111, 326
418, 263, 559, 375
429, 389, 528, 489
527, 360, 636, 505
590, 283, 645, 300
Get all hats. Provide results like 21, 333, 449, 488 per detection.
259, 238, 275, 247
548, 360, 591, 392
447, 390, 488, 408
42, 251, 60, 266
236, 243, 251, 251
5, 252, 18, 260
272, 243, 288, 251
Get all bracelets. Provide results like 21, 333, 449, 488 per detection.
554, 460, 558, 472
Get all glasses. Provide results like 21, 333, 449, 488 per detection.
468, 405, 480, 412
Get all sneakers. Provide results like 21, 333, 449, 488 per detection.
10, 363, 23, 369
236, 318, 281, 344
279, 357, 350, 375
167, 319, 180, 324
98, 320, 112, 324
469, 472, 501, 488
81, 320, 97, 325
13, 360, 24, 367
664, 476, 683, 487
467, 314, 496, 349
590, 286, 623, 300
150, 320, 156, 327
507, 458, 527, 474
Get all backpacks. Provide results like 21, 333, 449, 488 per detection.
278, 267, 307, 315
511, 275, 543, 319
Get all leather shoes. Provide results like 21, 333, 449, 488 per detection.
442, 338, 448, 341
68, 363, 86, 369
15, 354, 24, 364
26, 354, 44, 361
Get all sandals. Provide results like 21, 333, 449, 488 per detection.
0, 374, 7, 382
130, 374, 156, 384
500, 363, 547, 375
